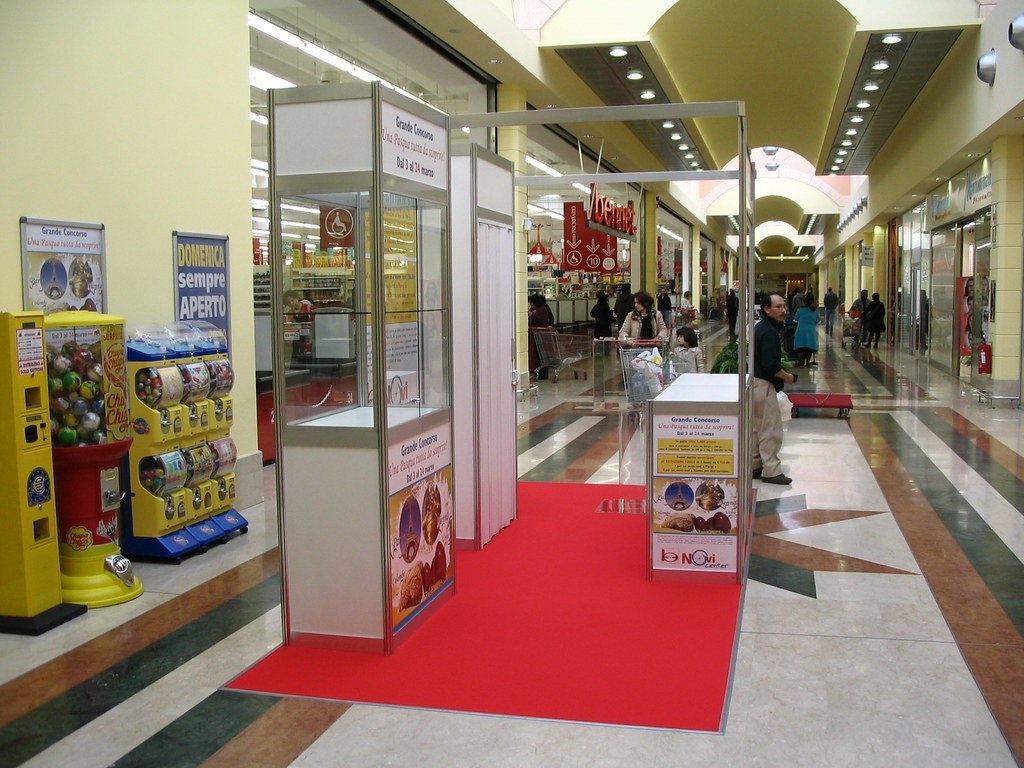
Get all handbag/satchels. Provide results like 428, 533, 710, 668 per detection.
630, 347, 664, 373
609, 310, 616, 324
734, 297, 737, 307
776, 391, 794, 421
850, 309, 861, 318
866, 311, 873, 320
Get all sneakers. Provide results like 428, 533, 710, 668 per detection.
762, 473, 792, 483
753, 467, 762, 478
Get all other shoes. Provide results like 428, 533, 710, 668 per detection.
796, 360, 805, 367
874, 345, 877, 348
806, 361, 811, 367
865, 344, 871, 347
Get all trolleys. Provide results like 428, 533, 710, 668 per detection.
614, 340, 692, 419
788, 392, 853, 420
840, 311, 862, 347
530, 326, 591, 382
708, 297, 727, 318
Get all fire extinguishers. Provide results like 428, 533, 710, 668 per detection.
977, 331, 991, 374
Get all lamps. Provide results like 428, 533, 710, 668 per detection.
855, 99, 871, 109
627, 70, 643, 80
831, 129, 857, 171
850, 116, 864, 122
640, 89, 655, 100
871, 59, 891, 71
662, 121, 674, 129
671, 134, 699, 167
862, 82, 879, 91
880, 34, 902, 44
766, 160, 779, 172
763, 147, 778, 155
609, 46, 628, 57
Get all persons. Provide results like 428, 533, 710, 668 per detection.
670, 325, 705, 373
726, 290, 739, 335
528, 294, 555, 379
590, 295, 614, 357
282, 290, 317, 354
681, 291, 695, 310
964, 277, 989, 349
752, 293, 798, 484
657, 288, 672, 330
921, 290, 929, 350
619, 290, 668, 346
614, 283, 635, 354
791, 288, 821, 368
289, 291, 316, 370
849, 290, 885, 348
824, 287, 839, 334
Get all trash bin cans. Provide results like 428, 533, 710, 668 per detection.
783, 326, 799, 362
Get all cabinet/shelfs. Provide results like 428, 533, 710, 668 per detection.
265, 83, 455, 655
644, 373, 749, 585
254, 263, 355, 307
